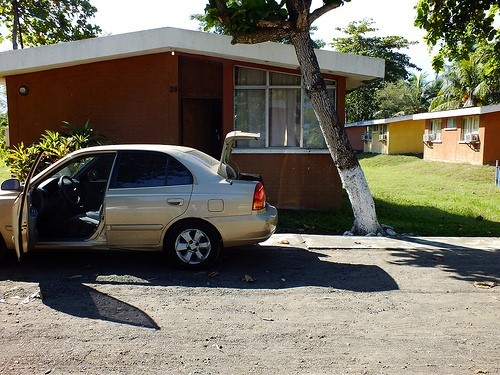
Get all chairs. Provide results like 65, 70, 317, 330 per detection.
75, 158, 136, 226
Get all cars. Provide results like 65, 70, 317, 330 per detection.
1, 130, 281, 271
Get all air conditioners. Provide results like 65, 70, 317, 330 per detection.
361, 135, 369, 140
465, 133, 479, 142
423, 134, 433, 141
379, 134, 387, 140
